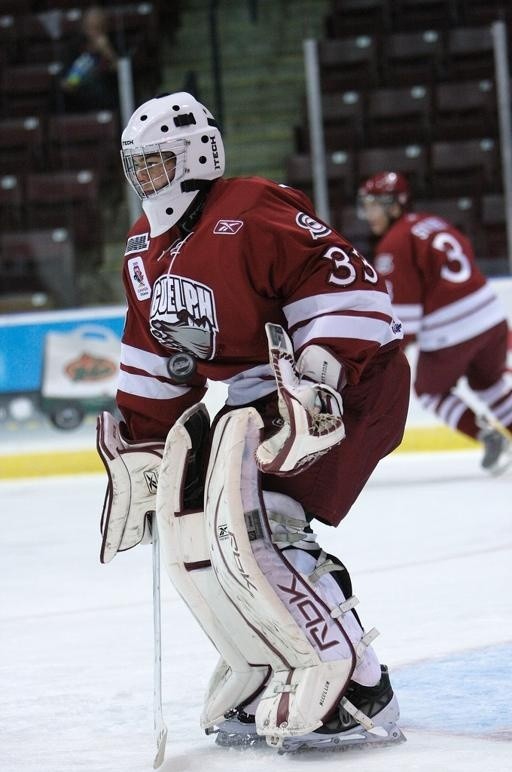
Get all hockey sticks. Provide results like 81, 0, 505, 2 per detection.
153, 511, 168, 769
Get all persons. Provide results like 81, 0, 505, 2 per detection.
355, 169, 512, 467
44, 5, 124, 115
92, 87, 415, 741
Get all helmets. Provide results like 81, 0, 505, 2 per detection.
119, 95, 225, 238
356, 172, 411, 219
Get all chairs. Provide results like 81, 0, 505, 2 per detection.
0, 1, 146, 301
294, 4, 511, 274
160, 296, 160, 299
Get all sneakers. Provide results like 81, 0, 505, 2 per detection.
476, 416, 505, 467
215, 710, 259, 734
287, 667, 400, 740
